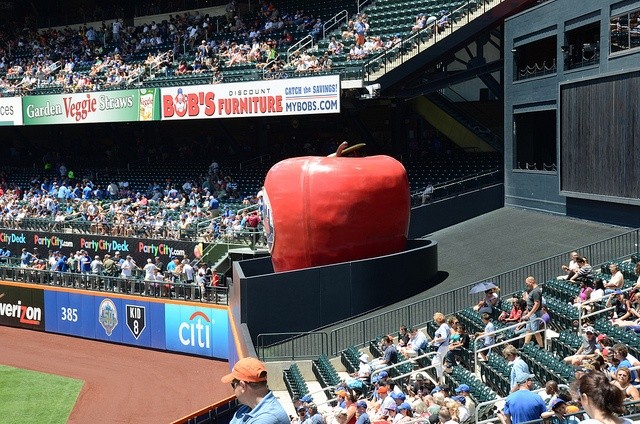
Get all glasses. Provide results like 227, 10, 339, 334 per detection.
617, 373, 629, 378
298, 410, 306, 413
513, 301, 518, 304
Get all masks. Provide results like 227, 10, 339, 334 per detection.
299, 413, 308, 416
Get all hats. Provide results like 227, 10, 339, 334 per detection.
359, 354, 370, 364
455, 384, 470, 393
398, 403, 411, 410
335, 387, 346, 391
585, 325, 595, 335
551, 399, 568, 411
392, 393, 407, 401
292, 395, 301, 402
429, 413, 440, 423
613, 290, 623, 295
595, 333, 607, 343
298, 405, 305, 412
372, 375, 380, 383
516, 372, 536, 386
335, 390, 346, 397
376, 386, 387, 395
299, 396, 312, 402
430, 387, 441, 396
452, 396, 466, 405
352, 388, 363, 394
387, 335, 393, 341
377, 371, 388, 379
416, 373, 424, 381
351, 401, 367, 409
220, 356, 269, 383
385, 404, 398, 412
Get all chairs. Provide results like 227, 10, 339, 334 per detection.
420, 346, 437, 373
551, 330, 583, 356
30, 44, 189, 95
445, 366, 497, 411
144, 1, 365, 89
370, 335, 413, 378
426, 318, 479, 370
493, 302, 512, 320
520, 341, 578, 385
540, 293, 578, 333
456, 307, 513, 342
283, 363, 314, 405
592, 319, 637, 355
601, 259, 636, 278
340, 346, 364, 371
284, 0, 484, 80
479, 352, 539, 396
542, 278, 582, 304
312, 355, 345, 399
595, 273, 634, 294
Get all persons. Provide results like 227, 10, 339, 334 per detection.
1, 159, 265, 301
220, 356, 291, 424
420, 182, 434, 206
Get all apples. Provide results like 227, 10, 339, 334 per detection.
262, 142, 412, 272
